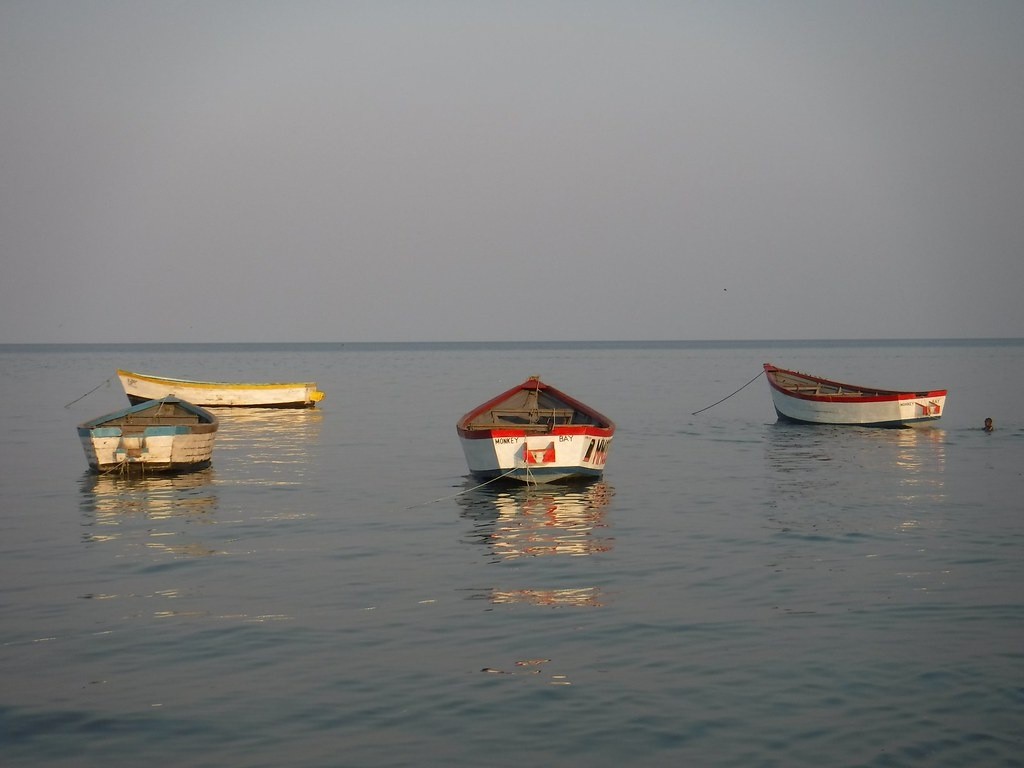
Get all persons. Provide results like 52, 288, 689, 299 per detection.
981, 417, 993, 432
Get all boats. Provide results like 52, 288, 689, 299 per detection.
115, 366, 325, 407
456, 375, 616, 485
76, 394, 219, 473
763, 363, 948, 427
